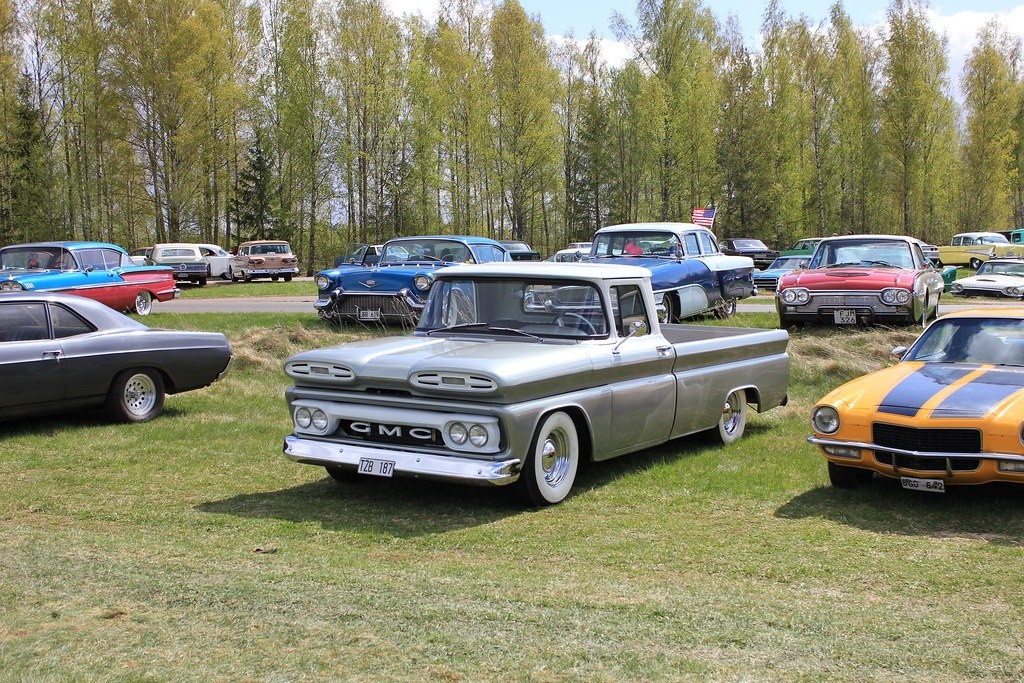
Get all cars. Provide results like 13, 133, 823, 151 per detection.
231, 238, 300, 284
334, 244, 412, 268
950, 253, 1024, 302
0, 241, 180, 317
749, 255, 821, 290
808, 306, 1024, 495
541, 222, 759, 325
314, 235, 514, 326
118, 243, 235, 287
778, 238, 824, 257
868, 227, 1024, 292
495, 240, 542, 262
0, 290, 235, 424
775, 233, 944, 330
716, 237, 779, 260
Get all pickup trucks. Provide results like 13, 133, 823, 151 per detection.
282, 259, 792, 506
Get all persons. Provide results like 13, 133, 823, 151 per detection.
847, 230, 855, 235
832, 233, 838, 236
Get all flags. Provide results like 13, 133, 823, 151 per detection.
692, 209, 715, 225
